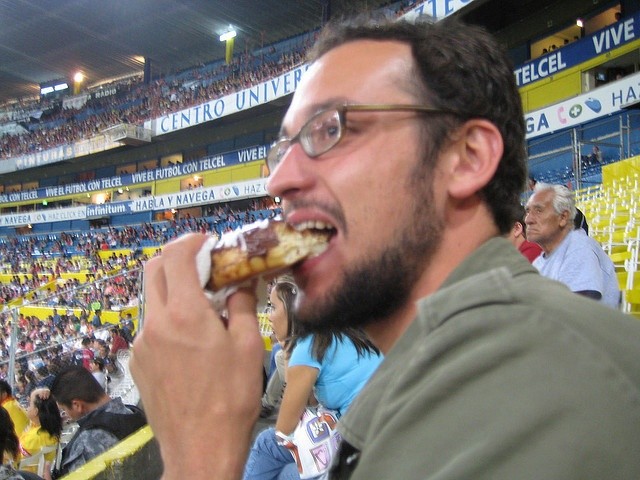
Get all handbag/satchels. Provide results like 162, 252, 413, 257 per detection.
275, 404, 343, 479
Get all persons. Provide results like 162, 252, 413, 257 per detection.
258, 349, 287, 418
527, 175, 535, 190
13, 388, 62, 480
128, 11, 640, 480
265, 343, 283, 391
0, 406, 45, 479
573, 146, 603, 166
0, 378, 32, 435
47, 365, 147, 476
244, 278, 382, 480
525, 181, 619, 310
505, 204, 543, 264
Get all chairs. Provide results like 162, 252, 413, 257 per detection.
526, 151, 640, 320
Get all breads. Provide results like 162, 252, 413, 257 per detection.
195, 216, 332, 302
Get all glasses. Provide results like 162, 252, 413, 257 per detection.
267, 104, 468, 175
